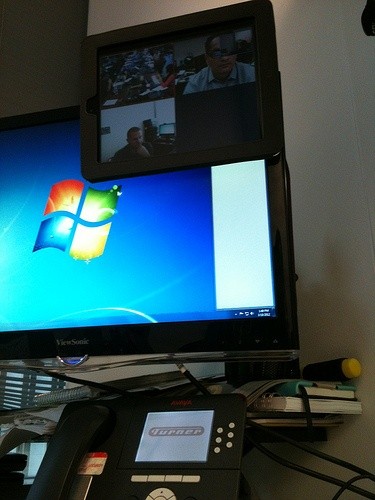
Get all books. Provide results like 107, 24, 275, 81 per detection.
1, 366, 364, 433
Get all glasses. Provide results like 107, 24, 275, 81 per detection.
208, 48, 238, 57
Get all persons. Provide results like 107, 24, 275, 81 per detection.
184, 56, 200, 73
183, 34, 256, 93
235, 39, 253, 65
113, 126, 155, 161
102, 45, 174, 103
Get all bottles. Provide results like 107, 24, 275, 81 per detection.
303, 358, 361, 383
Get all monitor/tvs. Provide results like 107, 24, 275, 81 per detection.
79, 0, 284, 183
0, 105, 300, 359
